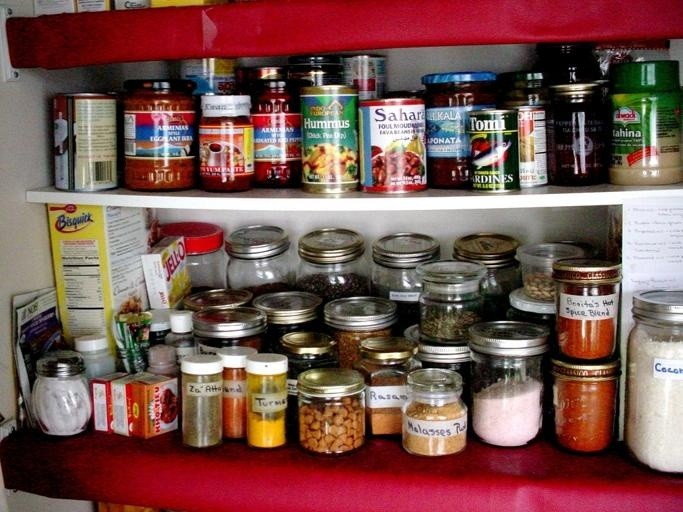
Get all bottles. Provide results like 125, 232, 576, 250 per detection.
200, 96, 253, 192
225, 225, 297, 296
610, 61, 682, 185
372, 233, 441, 319
217, 346, 256, 441
296, 368, 366, 456
297, 229, 371, 303
245, 351, 288, 449
471, 319, 548, 448
537, 42, 602, 86
551, 84, 608, 185
33, 352, 92, 437
279, 332, 340, 436
390, 90, 423, 99
356, 337, 422, 438
469, 108, 520, 193
142, 309, 177, 346
182, 59, 236, 95
555, 257, 623, 364
147, 345, 180, 379
165, 312, 198, 364
159, 223, 226, 291
253, 291, 323, 354
301, 83, 359, 195
551, 353, 620, 455
404, 323, 474, 392
454, 233, 521, 293
341, 55, 386, 103
357, 97, 426, 194
184, 289, 252, 313
553, 241, 602, 265
193, 305, 266, 356
121, 80, 199, 190
497, 72, 551, 188
505, 286, 557, 333
416, 258, 491, 345
400, 368, 468, 457
624, 289, 683, 474
74, 334, 109, 377
288, 56, 341, 108
596, 41, 672, 82
181, 356, 223, 448
323, 295, 398, 368
242, 66, 301, 186
422, 71, 496, 189
52, 93, 120, 192
